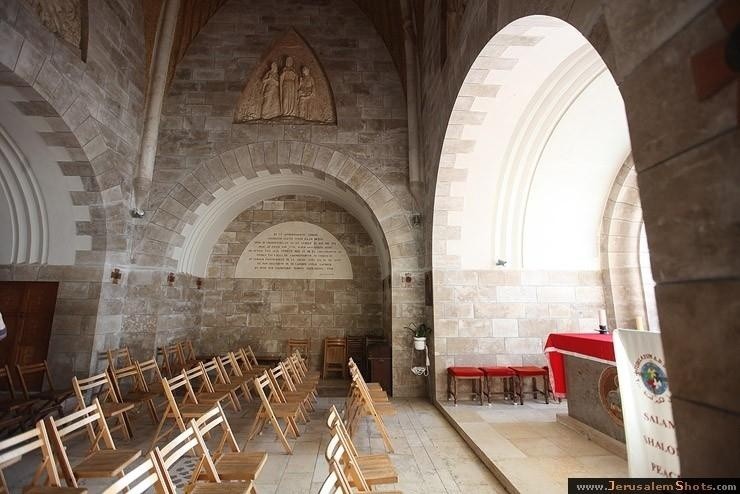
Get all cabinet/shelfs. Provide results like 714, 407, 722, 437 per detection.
0, 280, 59, 392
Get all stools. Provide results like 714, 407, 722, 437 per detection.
544, 366, 562, 403
507, 366, 549, 405
479, 367, 518, 407
447, 367, 484, 407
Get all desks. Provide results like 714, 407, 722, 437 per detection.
544, 332, 615, 400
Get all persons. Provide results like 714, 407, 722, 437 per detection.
260, 61, 281, 119
278, 55, 299, 118
295, 65, 315, 120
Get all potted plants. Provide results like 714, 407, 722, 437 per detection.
403, 321, 432, 350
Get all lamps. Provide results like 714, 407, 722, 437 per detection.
413, 215, 420, 227
132, 207, 145, 218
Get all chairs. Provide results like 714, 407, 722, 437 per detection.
0, 334, 403, 494
